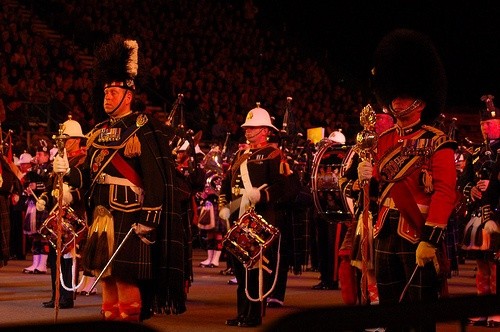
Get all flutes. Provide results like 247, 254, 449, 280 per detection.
456, 132, 492, 214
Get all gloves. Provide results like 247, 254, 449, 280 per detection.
52, 147, 71, 175
416, 241, 436, 266
136, 223, 153, 234
358, 162, 373, 185
246, 187, 261, 204
219, 208, 231, 219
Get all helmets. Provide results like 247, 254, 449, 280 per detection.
240, 103, 279, 132
13, 145, 58, 167
328, 129, 345, 144
56, 116, 87, 138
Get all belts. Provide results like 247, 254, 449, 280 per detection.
95, 173, 136, 186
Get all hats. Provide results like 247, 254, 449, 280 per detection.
480, 95, 500, 121
375, 28, 447, 121
179, 141, 206, 156
96, 34, 139, 92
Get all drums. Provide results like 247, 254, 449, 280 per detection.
37, 205, 88, 257
310, 139, 363, 225
220, 207, 280, 269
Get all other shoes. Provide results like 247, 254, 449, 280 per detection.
313, 281, 337, 291
226, 315, 260, 327
228, 278, 237, 285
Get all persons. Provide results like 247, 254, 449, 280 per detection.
219, 102, 299, 326
0, 0, 152, 149
358, 96, 458, 332
0, 129, 23, 266
53, 40, 165, 321
44, 114, 87, 308
307, 128, 351, 290
11, 139, 55, 273
200, 145, 238, 284
460, 94, 500, 323
59, 0, 374, 145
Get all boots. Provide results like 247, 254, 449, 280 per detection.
23, 255, 40, 274
77, 276, 97, 295
34, 254, 48, 275
200, 249, 222, 268
42, 258, 73, 308
